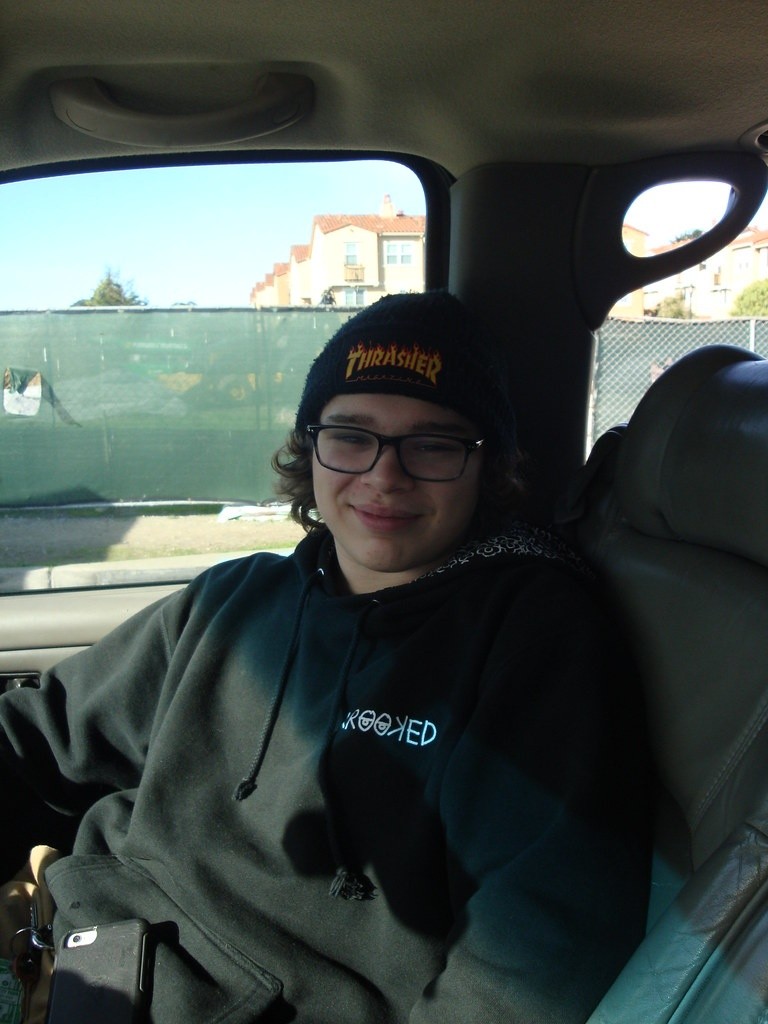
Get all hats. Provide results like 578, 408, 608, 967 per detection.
292, 293, 509, 453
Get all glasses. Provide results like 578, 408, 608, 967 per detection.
303, 421, 490, 482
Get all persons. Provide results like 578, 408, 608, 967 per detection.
1, 292, 652, 1024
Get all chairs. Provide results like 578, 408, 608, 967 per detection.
588, 802, 767, 1024
578, 339, 768, 937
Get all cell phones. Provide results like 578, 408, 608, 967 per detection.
45, 919, 151, 1024
